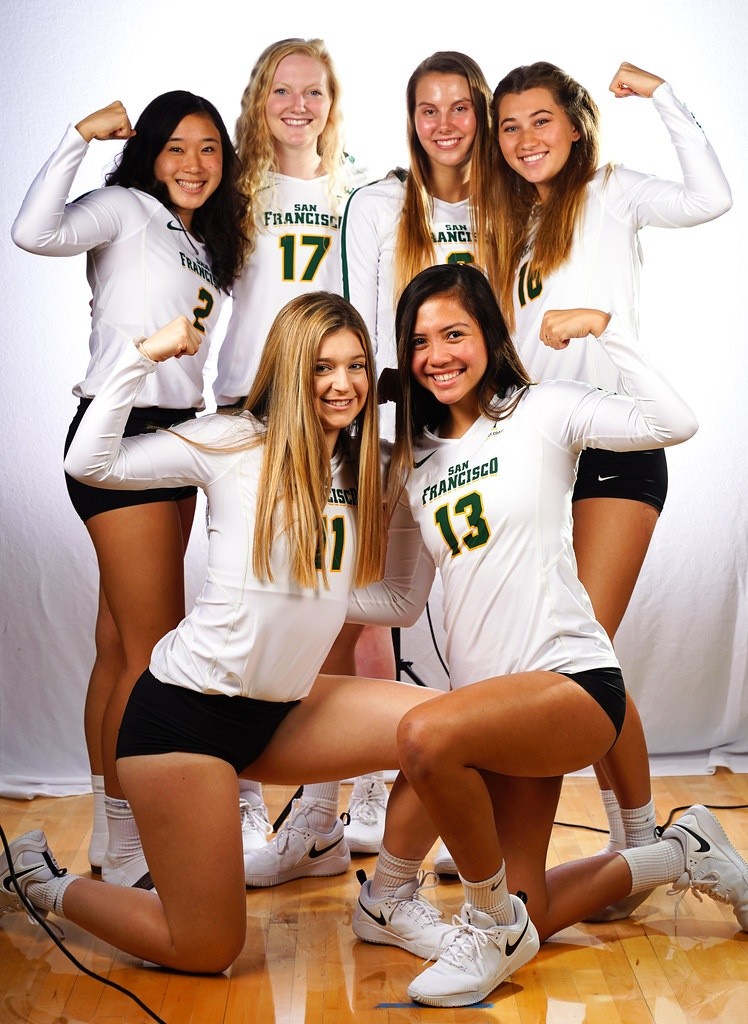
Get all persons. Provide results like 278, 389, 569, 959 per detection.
0, 37, 748, 1008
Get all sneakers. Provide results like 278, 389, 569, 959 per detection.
339, 776, 389, 852
408, 889, 538, 1008
654, 803, 748, 931
354, 867, 461, 960
580, 888, 655, 924
237, 789, 275, 855
0, 829, 68, 940
237, 798, 347, 886
434, 842, 460, 875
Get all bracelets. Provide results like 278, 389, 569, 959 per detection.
139, 342, 154, 362
